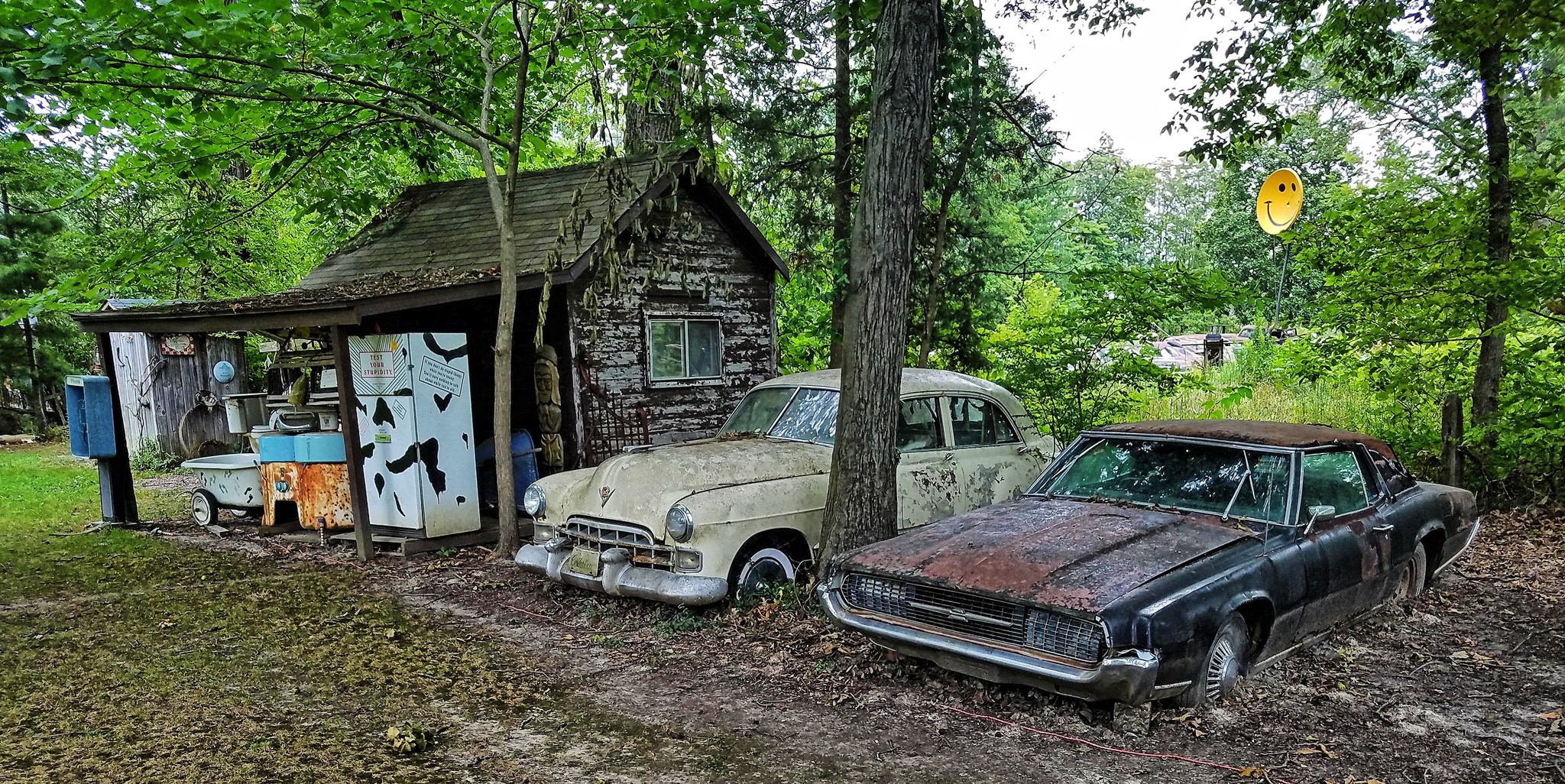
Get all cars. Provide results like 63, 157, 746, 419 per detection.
816, 421, 1481, 710
514, 367, 1063, 604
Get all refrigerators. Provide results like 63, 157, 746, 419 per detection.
348, 333, 481, 539
